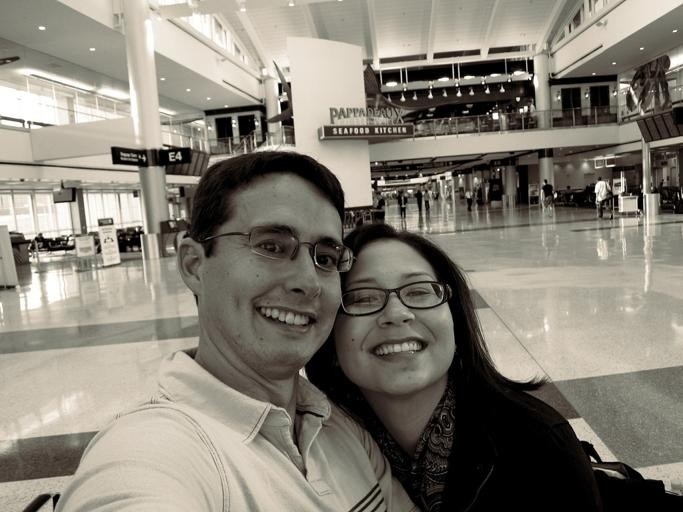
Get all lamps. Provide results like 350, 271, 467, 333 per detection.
388, 83, 505, 103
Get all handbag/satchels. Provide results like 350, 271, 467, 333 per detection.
606, 191, 613, 199
552, 191, 558, 200
582, 441, 683, 510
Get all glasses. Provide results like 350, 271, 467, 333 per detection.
200, 226, 357, 272
335, 280, 454, 316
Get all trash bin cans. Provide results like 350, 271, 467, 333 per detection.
645, 193, 660, 215
140, 234, 160, 260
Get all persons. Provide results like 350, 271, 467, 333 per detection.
527, 101, 536, 122
373, 177, 613, 220
304, 225, 683, 512
53, 151, 423, 512
